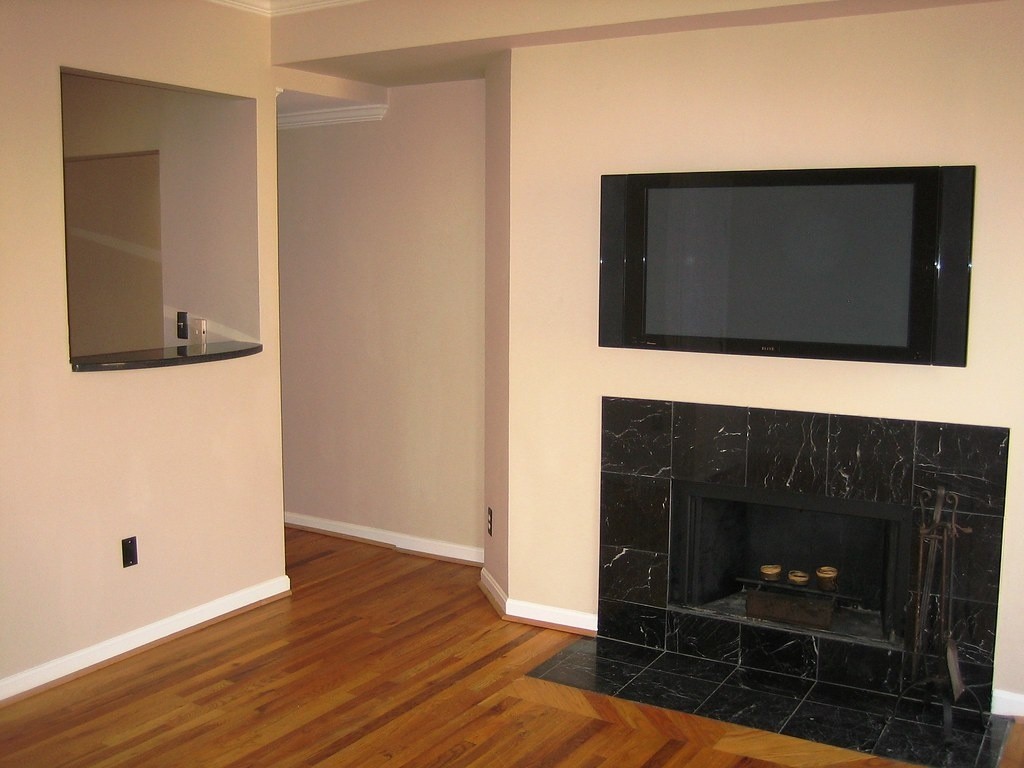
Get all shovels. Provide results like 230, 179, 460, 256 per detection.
945, 493, 966, 702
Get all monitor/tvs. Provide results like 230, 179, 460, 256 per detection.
597, 166, 977, 368
